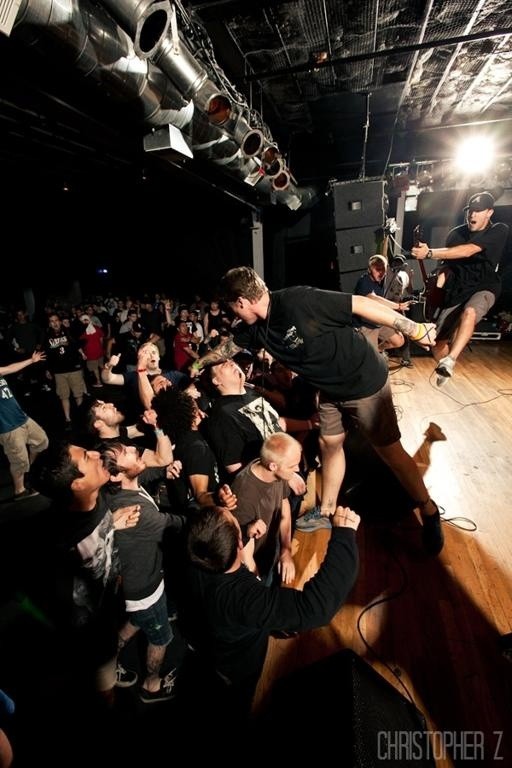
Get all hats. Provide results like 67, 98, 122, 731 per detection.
133, 321, 143, 332
81, 315, 89, 321
463, 193, 495, 211
398, 270, 409, 294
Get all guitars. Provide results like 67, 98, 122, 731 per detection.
413, 226, 449, 323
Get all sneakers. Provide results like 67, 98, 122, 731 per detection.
114, 663, 138, 688
14, 488, 40, 501
435, 363, 453, 387
138, 661, 177, 704
421, 499, 443, 555
380, 351, 390, 367
296, 505, 333, 532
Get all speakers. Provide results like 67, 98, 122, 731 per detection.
334, 225, 385, 274
339, 264, 389, 293
331, 180, 385, 230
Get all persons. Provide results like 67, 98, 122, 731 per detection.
2, 191, 511, 768
